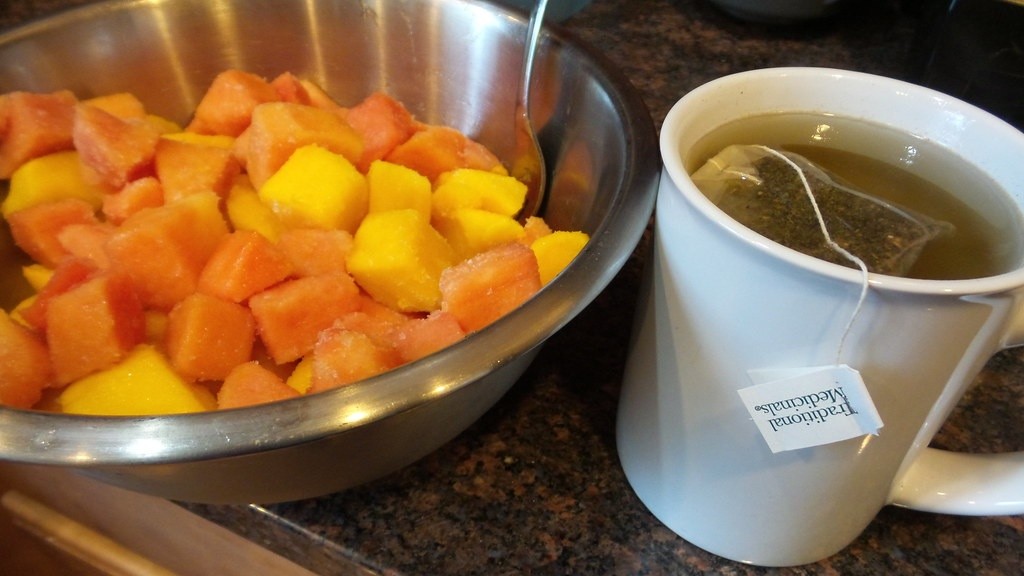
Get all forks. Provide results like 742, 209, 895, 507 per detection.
510, 0, 548, 226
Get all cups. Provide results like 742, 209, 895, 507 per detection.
616, 67, 1024, 567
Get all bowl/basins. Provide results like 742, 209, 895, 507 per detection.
0, 0, 662, 505
